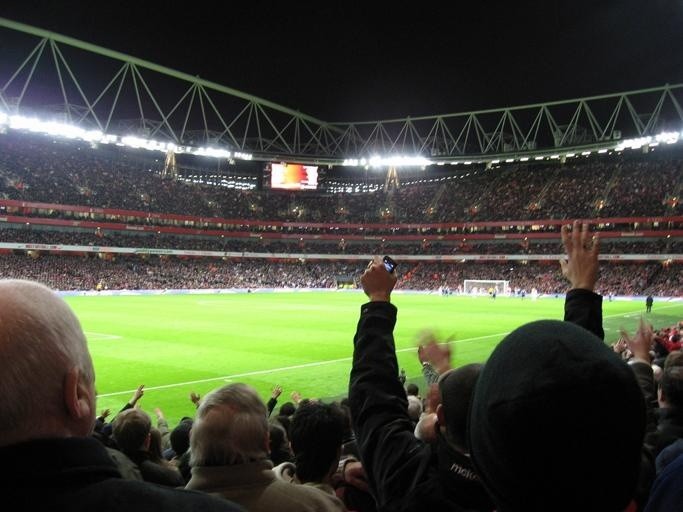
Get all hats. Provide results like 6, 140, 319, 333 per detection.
439, 362, 481, 456
469, 320, 645, 511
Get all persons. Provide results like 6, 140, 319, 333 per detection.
1, 123, 683, 512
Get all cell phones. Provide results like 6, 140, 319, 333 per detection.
382, 255, 397, 274
